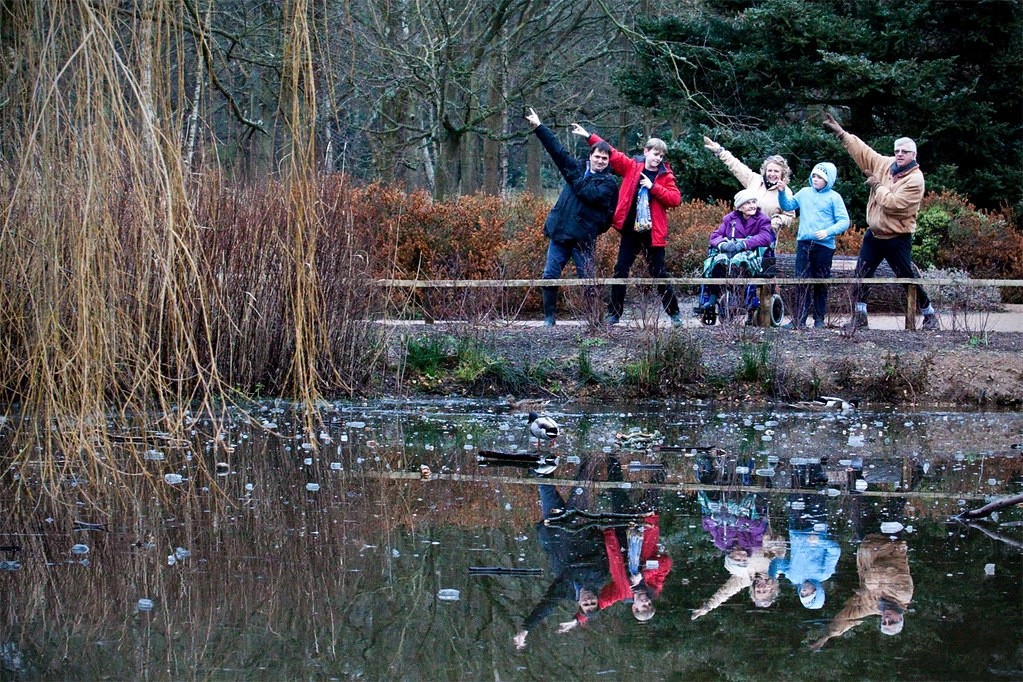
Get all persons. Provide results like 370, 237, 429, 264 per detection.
571, 123, 683, 328
703, 137, 796, 239
689, 456, 913, 649
696, 190, 773, 312
821, 113, 941, 332
527, 108, 621, 327
515, 464, 673, 651
776, 162, 850, 328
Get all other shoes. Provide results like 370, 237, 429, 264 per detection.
814, 319, 824, 328
607, 315, 620, 323
843, 311, 869, 329
781, 321, 806, 329
543, 317, 556, 326
922, 314, 940, 330
670, 311, 683, 327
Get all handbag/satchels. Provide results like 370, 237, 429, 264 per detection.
626, 521, 644, 577
634, 186, 653, 232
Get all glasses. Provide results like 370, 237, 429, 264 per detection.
880, 621, 899, 626
893, 149, 913, 154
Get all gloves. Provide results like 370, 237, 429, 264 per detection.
712, 507, 737, 525
808, 633, 829, 651
563, 167, 580, 184
690, 605, 707, 621
863, 169, 880, 188
822, 113, 844, 139
725, 240, 746, 259
703, 136, 720, 153
770, 216, 782, 229
718, 241, 735, 252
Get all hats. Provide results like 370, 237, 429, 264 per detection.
723, 554, 749, 576
799, 592, 817, 608
733, 189, 758, 210
811, 163, 827, 183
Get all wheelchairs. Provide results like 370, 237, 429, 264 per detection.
693, 226, 784, 328
